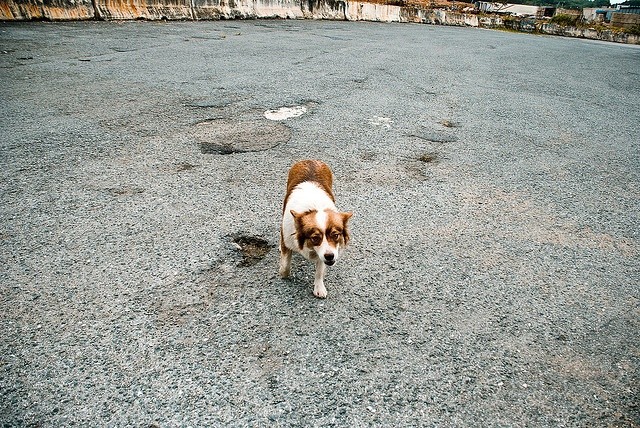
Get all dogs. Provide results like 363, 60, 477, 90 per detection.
279, 158, 353, 298
